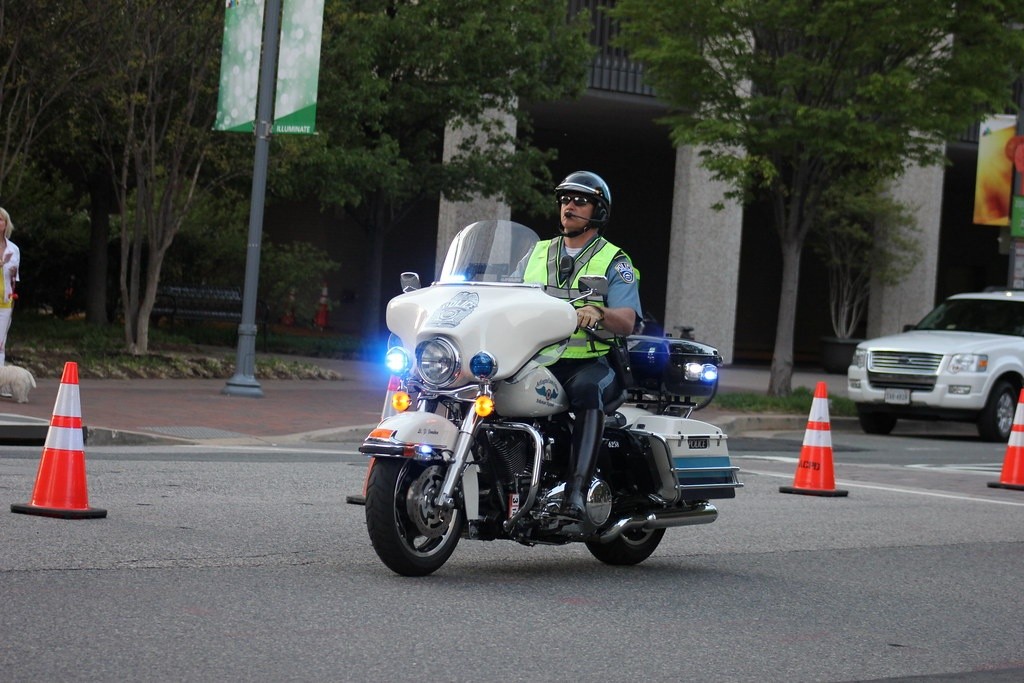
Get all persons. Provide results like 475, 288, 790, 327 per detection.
0, 206, 20, 397
506, 170, 644, 521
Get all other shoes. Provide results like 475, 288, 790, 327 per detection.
0, 392, 12, 397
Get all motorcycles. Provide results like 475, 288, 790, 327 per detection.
345, 218, 747, 579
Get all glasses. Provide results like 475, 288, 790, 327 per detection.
559, 195, 590, 206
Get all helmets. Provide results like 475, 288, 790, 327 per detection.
554, 171, 612, 229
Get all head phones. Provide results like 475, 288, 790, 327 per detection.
590, 201, 608, 229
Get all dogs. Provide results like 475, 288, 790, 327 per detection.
0, 365, 37, 403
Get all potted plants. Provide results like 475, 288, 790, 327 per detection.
783, 168, 923, 375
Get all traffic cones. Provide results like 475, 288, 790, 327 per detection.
344, 373, 401, 506
275, 286, 296, 327
985, 388, 1024, 493
309, 285, 333, 331
9, 361, 110, 520
778, 381, 850, 497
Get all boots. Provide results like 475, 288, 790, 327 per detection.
559, 408, 605, 521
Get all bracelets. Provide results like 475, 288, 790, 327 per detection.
581, 301, 605, 320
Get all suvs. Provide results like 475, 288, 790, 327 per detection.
847, 285, 1024, 444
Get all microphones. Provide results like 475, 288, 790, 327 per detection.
564, 212, 606, 225
